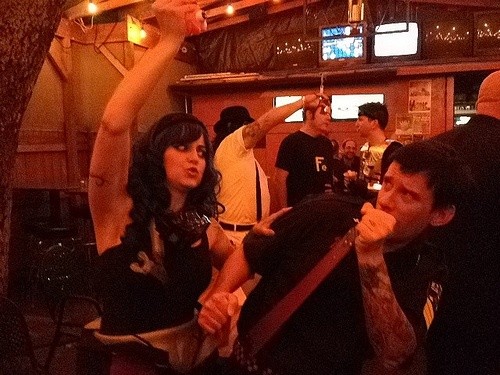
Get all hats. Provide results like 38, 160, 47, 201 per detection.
213, 106, 255, 133
475, 69, 500, 109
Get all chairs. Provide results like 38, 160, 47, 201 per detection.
0, 245, 102, 375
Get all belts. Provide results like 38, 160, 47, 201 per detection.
218, 222, 252, 231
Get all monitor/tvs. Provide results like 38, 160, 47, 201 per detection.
317, 21, 368, 67
370, 20, 422, 62
274, 96, 306, 123
330, 93, 385, 121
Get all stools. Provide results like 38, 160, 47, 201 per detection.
23, 218, 75, 290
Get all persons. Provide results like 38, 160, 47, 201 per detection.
88, 0, 292, 375
426, 69, 500, 375
198, 139, 474, 375
330, 139, 353, 191
341, 139, 361, 178
198, 93, 329, 355
270, 96, 333, 217
356, 103, 391, 189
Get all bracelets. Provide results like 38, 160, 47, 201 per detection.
314, 91, 318, 97
